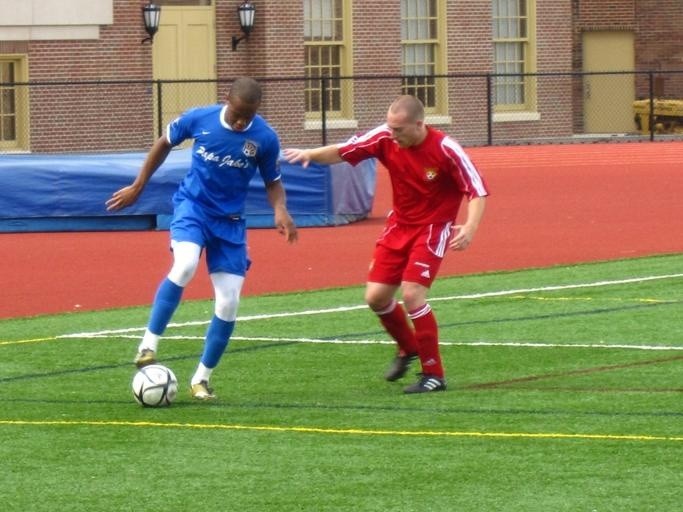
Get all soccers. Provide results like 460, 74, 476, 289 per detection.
132, 364, 177, 407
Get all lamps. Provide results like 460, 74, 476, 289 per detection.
141, 0, 161, 44
231, 0, 255, 50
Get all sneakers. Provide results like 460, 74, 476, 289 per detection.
385, 349, 420, 381
190, 381, 216, 400
403, 377, 447, 394
133, 348, 156, 368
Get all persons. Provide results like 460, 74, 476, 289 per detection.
104, 78, 300, 403
280, 93, 490, 391
